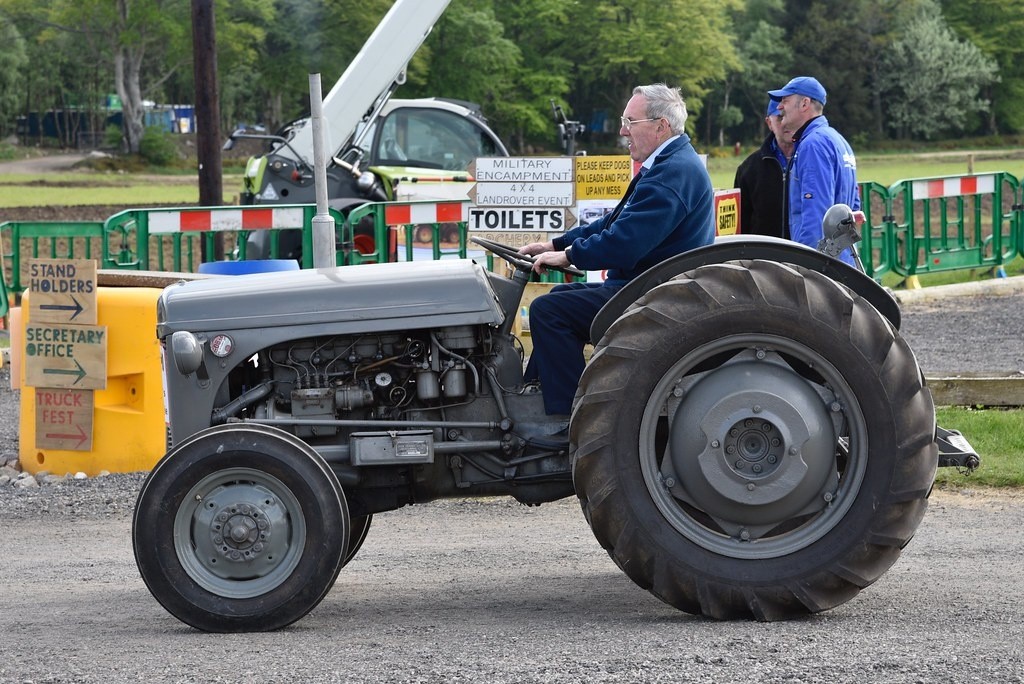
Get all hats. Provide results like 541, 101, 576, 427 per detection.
767, 99, 781, 116
768, 77, 827, 107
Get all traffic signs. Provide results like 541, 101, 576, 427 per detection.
34, 387, 92, 451
25, 325, 107, 390
29, 258, 97, 324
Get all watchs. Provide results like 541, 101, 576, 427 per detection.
565, 244, 574, 265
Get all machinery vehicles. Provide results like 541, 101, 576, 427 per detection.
129, 0, 982, 635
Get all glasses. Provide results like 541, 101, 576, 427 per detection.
620, 116, 670, 130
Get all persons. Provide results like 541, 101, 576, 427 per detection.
518, 83, 716, 440
768, 76, 860, 268
733, 99, 798, 241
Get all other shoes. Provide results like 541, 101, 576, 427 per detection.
514, 419, 568, 449
